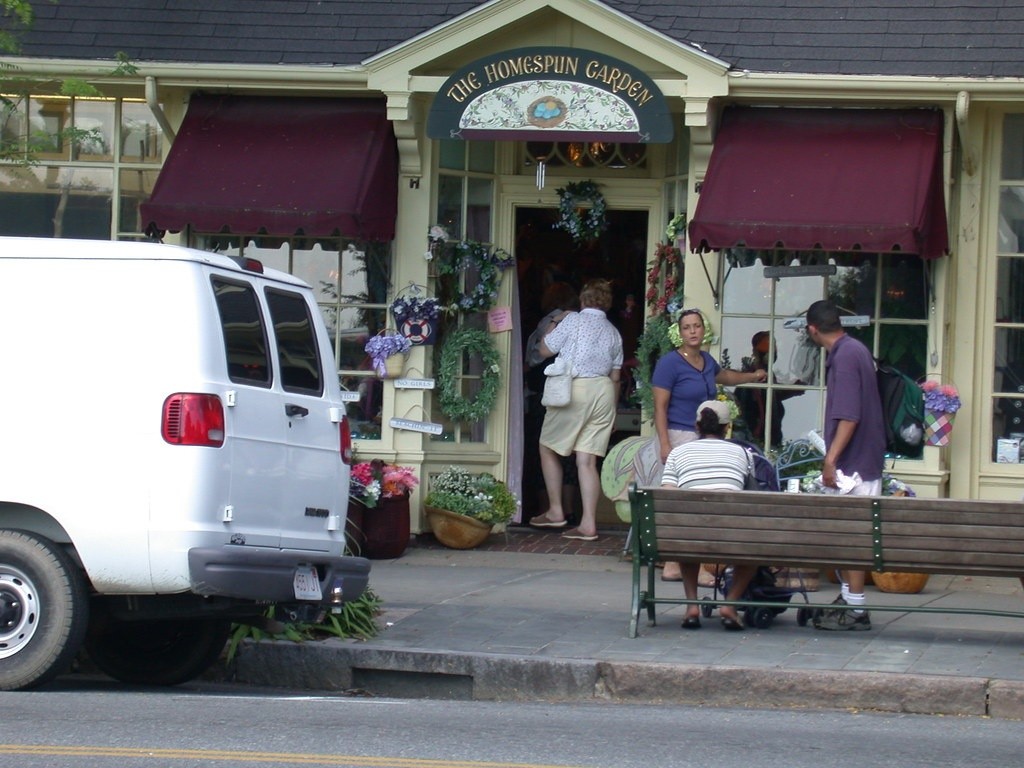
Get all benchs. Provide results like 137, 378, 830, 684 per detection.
619, 487, 1024, 639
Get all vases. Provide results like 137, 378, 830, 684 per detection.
395, 314, 438, 346
423, 503, 494, 550
380, 351, 405, 379
346, 492, 412, 559
923, 404, 956, 447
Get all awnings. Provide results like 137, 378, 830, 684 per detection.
689, 107, 949, 262
138, 97, 397, 237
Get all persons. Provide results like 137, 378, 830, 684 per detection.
804, 301, 887, 631
512, 242, 645, 542
651, 302, 767, 588
661, 400, 756, 631
731, 332, 805, 447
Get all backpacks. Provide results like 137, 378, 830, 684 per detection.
874, 355, 926, 459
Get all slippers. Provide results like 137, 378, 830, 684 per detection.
721, 614, 744, 629
697, 573, 724, 588
562, 528, 598, 540
681, 615, 701, 627
530, 513, 567, 526
662, 575, 685, 581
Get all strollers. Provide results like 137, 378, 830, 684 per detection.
703, 439, 825, 628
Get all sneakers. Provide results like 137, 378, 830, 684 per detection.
814, 608, 870, 629
814, 593, 847, 616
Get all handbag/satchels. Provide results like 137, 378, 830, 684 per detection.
541, 361, 574, 407
374, 328, 404, 378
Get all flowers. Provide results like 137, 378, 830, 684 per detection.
664, 214, 687, 243
440, 240, 518, 314
351, 459, 418, 498
392, 293, 439, 320
366, 336, 412, 378
922, 381, 961, 414
552, 178, 608, 243
647, 242, 680, 311
422, 222, 449, 260
422, 467, 515, 524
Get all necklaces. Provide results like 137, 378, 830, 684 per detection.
680, 346, 696, 363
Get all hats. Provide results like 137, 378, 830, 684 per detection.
696, 400, 732, 427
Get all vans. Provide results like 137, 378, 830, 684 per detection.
0, 231, 373, 695
